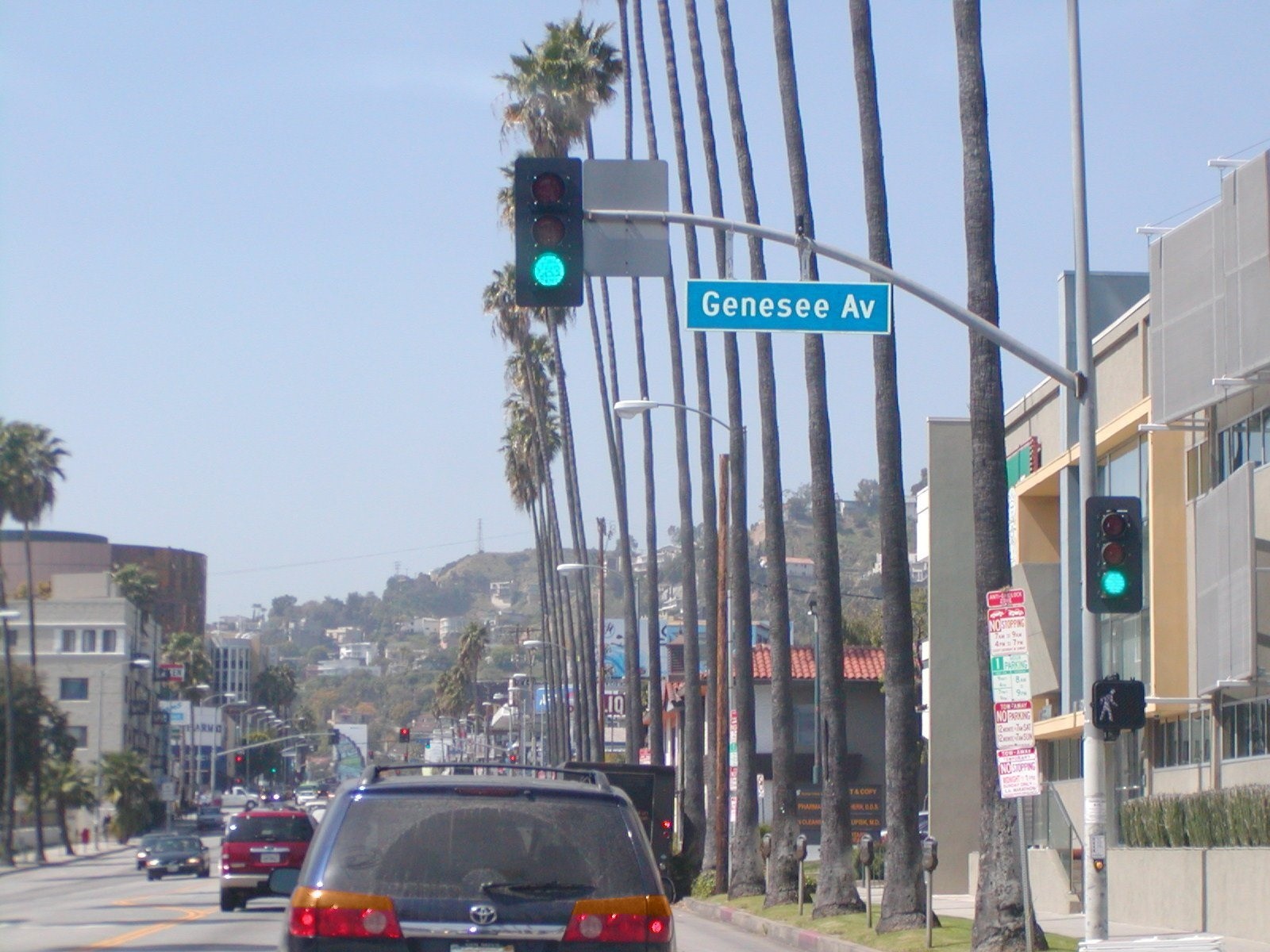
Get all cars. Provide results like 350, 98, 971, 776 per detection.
144, 835, 211, 881
135, 832, 177, 870
258, 780, 337, 823
197, 805, 223, 830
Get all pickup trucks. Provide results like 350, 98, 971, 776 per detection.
221, 786, 259, 811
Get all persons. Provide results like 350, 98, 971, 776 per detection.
81, 828, 89, 853
103, 812, 112, 842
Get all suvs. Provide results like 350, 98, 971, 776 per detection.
266, 760, 678, 952
219, 809, 320, 913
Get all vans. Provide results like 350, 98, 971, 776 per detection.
554, 760, 675, 882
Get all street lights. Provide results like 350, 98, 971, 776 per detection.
807, 598, 821, 785
195, 692, 238, 787
438, 673, 548, 778
556, 564, 640, 647
94, 658, 153, 851
211, 699, 247, 804
238, 705, 283, 757
615, 395, 749, 488
176, 683, 210, 812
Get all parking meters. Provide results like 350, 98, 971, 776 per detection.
922, 840, 939, 949
761, 832, 772, 896
795, 834, 807, 916
860, 834, 874, 929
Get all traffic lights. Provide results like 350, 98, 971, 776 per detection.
1085, 495, 1142, 615
235, 755, 245, 774
512, 157, 584, 306
399, 729, 409, 744
1091, 680, 1146, 732
425, 741, 430, 749
